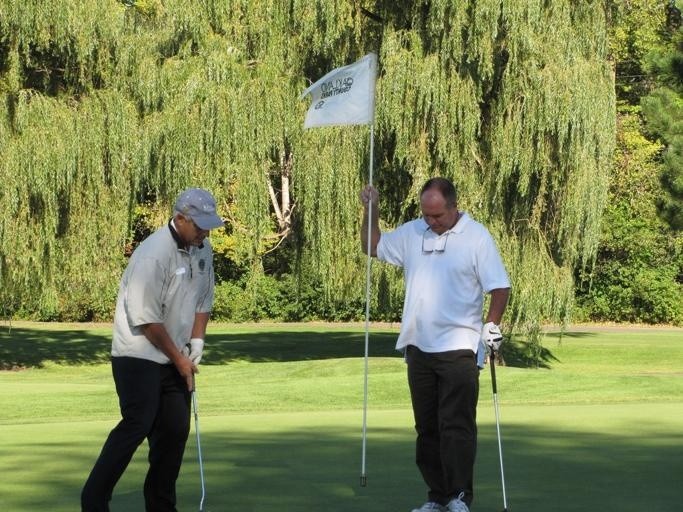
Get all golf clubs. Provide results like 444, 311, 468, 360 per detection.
182, 344, 206, 511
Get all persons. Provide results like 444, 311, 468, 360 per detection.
356, 175, 513, 511
77, 188, 225, 511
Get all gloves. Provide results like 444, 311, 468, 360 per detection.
188, 338, 204, 366
481, 323, 503, 351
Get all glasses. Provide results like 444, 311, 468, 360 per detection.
420, 225, 449, 253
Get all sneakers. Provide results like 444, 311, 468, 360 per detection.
80, 492, 110, 511
412, 500, 446, 512
448, 498, 470, 512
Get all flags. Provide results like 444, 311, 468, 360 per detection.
296, 54, 377, 130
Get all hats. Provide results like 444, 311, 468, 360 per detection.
172, 189, 224, 231
